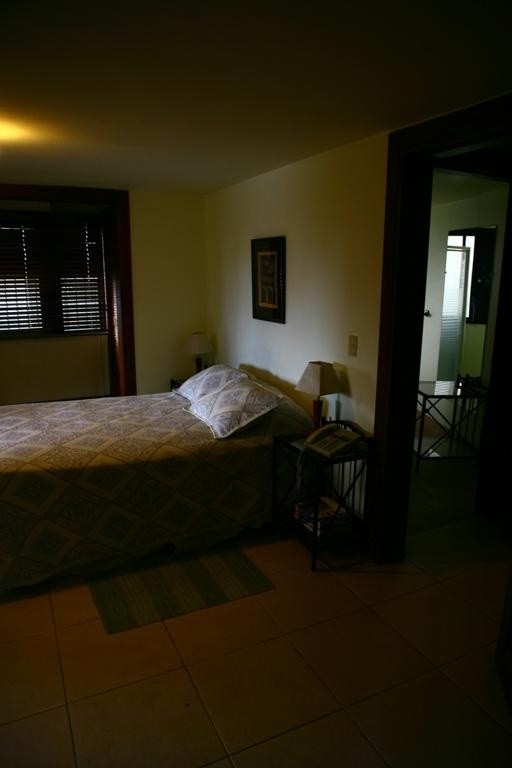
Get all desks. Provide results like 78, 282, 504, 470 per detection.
410, 373, 489, 473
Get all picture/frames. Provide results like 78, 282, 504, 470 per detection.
250, 235, 287, 324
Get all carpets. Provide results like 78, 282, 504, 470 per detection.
86, 546, 273, 635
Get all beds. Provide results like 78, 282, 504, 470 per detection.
1, 364, 328, 592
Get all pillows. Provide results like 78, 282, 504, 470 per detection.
183, 372, 281, 440
173, 359, 247, 403
227, 370, 314, 439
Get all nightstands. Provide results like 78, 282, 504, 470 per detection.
170, 378, 182, 390
271, 428, 385, 571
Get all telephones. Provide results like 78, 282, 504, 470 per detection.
302, 422, 361, 460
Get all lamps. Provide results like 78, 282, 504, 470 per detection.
293, 361, 344, 431
182, 329, 215, 373
